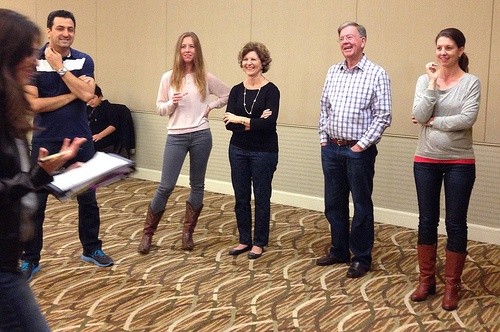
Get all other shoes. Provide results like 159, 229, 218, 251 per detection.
316, 254, 350, 266
247, 251, 261, 259
229, 244, 252, 255
345, 262, 368, 277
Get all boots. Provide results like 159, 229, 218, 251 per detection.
442, 248, 469, 311
138, 204, 165, 255
181, 201, 204, 249
411, 243, 438, 302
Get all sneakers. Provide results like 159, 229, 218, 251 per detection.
21, 260, 40, 282
82, 250, 114, 267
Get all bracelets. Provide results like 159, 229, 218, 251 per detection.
58, 67, 68, 76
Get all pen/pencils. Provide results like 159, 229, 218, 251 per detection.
36, 147, 83, 162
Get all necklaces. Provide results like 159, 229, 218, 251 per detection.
244, 79, 265, 115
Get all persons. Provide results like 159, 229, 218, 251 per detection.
137, 32, 231, 254
87, 84, 117, 152
316, 21, 391, 277
19, 10, 114, 280
0, 8, 87, 332
222, 41, 280, 259
410, 27, 482, 311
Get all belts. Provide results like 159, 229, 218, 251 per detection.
330, 138, 358, 147
